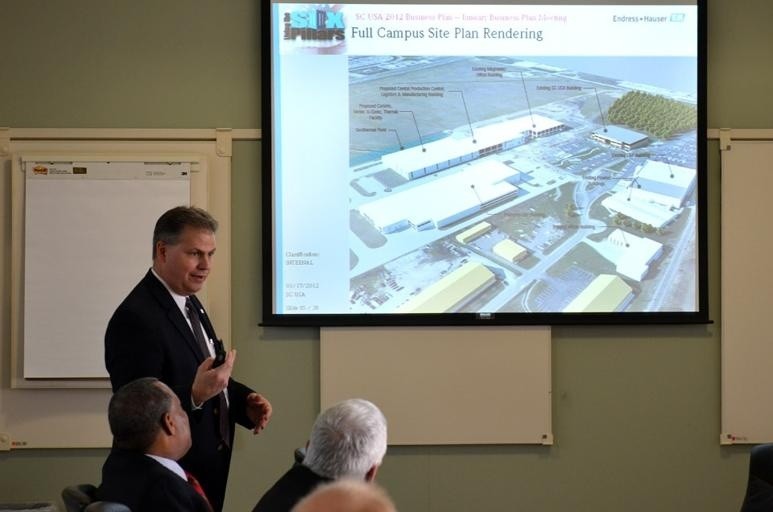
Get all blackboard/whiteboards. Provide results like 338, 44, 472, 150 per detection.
320, 327, 552, 444
0, 141, 231, 451
720, 128, 773, 446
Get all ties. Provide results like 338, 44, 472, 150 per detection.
184, 469, 213, 512
183, 297, 231, 448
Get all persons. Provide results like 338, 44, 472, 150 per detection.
104, 205, 273, 512
252, 398, 388, 512
290, 482, 396, 512
93, 377, 214, 512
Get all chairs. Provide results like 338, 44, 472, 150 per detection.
62, 482, 97, 511
85, 499, 131, 512
